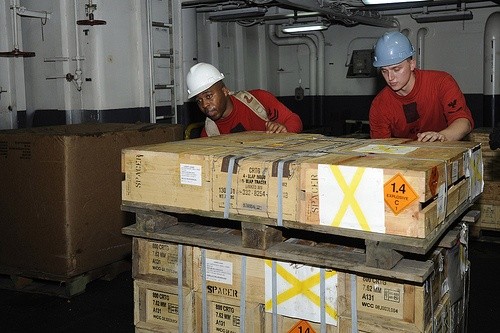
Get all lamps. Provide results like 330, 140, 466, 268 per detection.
281, 16, 328, 32
413, 3, 473, 23
209, 6, 265, 22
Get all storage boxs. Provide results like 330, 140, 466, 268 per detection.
0, 122, 500, 333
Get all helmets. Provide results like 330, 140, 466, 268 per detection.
373, 30, 416, 66
187, 62, 226, 98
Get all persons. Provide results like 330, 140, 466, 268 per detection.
368, 32, 474, 142
187, 63, 303, 138
